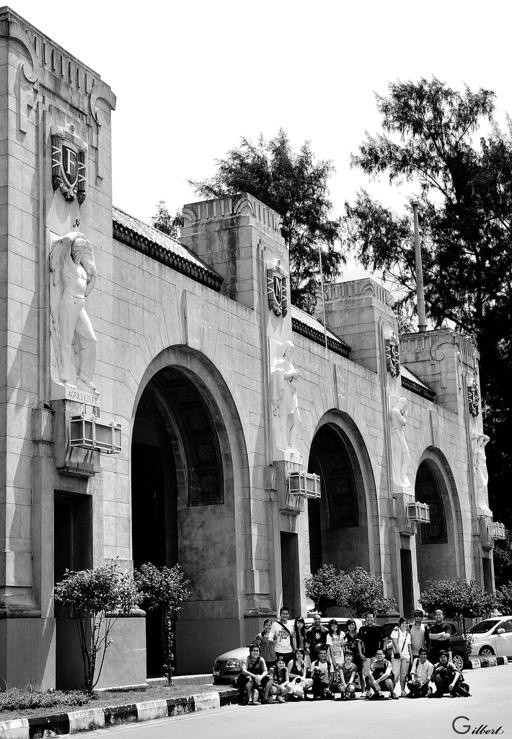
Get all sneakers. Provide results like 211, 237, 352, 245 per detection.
401, 692, 408, 697
360, 693, 366, 697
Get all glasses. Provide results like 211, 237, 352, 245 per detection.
314, 618, 320, 620
414, 614, 422, 617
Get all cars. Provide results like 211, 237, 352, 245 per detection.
378, 620, 468, 673
460, 614, 512, 657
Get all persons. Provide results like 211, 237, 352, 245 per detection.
471, 432, 491, 509
389, 396, 412, 488
235, 604, 469, 705
48, 231, 100, 393
269, 340, 302, 455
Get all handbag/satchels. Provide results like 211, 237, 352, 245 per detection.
394, 652, 400, 659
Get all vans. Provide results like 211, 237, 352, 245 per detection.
211, 614, 372, 686
